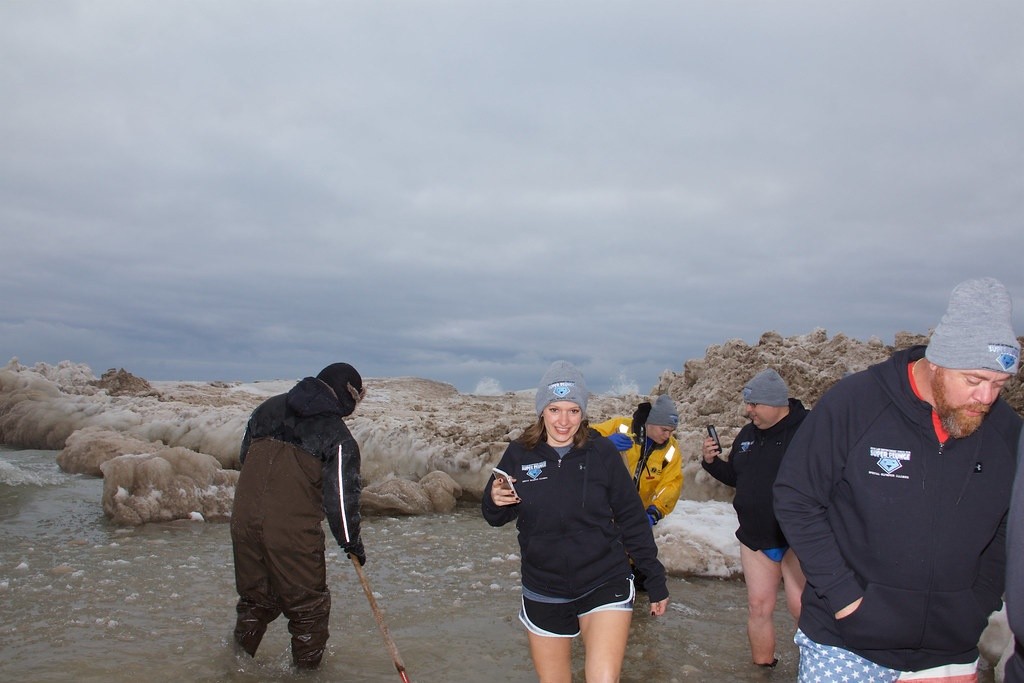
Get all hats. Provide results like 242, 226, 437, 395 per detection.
647, 394, 678, 426
926, 277, 1022, 371
536, 360, 588, 415
743, 368, 788, 407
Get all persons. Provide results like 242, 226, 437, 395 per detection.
587, 394, 684, 593
774, 277, 1024, 683
480, 362, 670, 682
230, 364, 365, 671
700, 369, 814, 670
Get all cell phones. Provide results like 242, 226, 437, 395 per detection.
707, 425, 722, 454
492, 467, 519, 499
